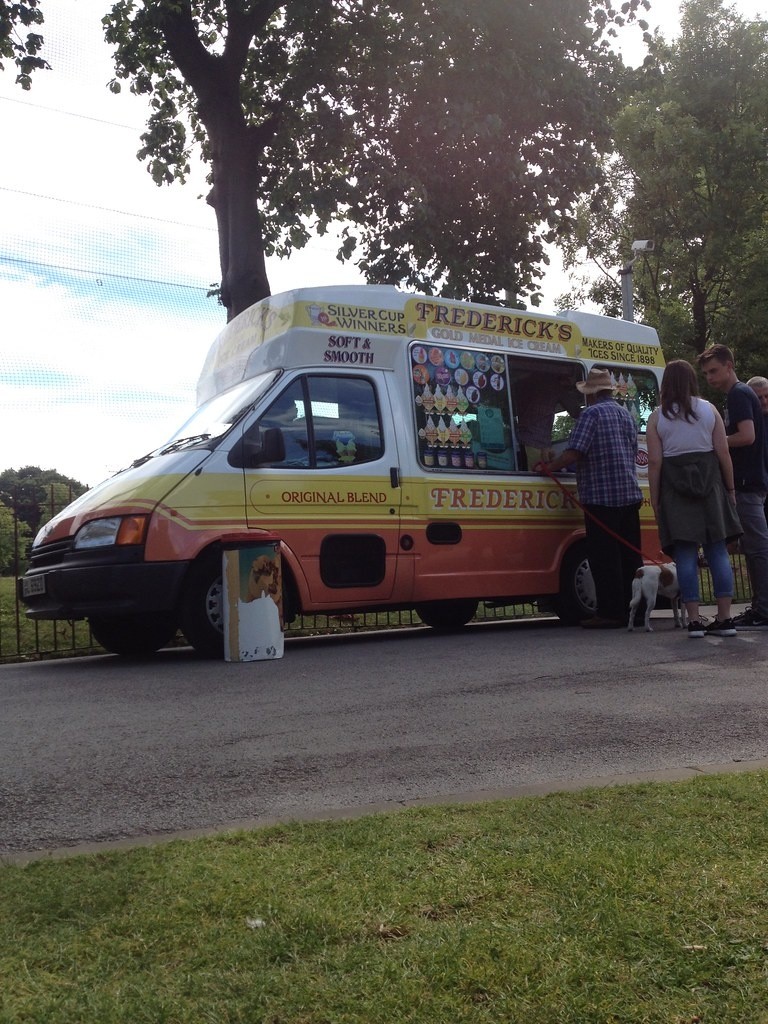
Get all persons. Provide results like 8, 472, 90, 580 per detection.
531, 370, 643, 629
516, 368, 584, 471
697, 344, 768, 631
646, 360, 745, 637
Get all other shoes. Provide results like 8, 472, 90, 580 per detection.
583, 616, 625, 627
626, 616, 645, 625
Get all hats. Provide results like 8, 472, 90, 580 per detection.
576, 368, 617, 394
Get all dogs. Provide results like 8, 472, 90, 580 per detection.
627, 562, 688, 631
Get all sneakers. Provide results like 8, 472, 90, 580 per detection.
732, 606, 768, 631
706, 615, 737, 635
688, 615, 708, 637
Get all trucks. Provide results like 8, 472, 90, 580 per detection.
18, 285, 670, 657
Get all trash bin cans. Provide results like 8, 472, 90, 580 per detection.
222, 536, 286, 662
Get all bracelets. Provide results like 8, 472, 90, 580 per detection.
726, 488, 735, 491
